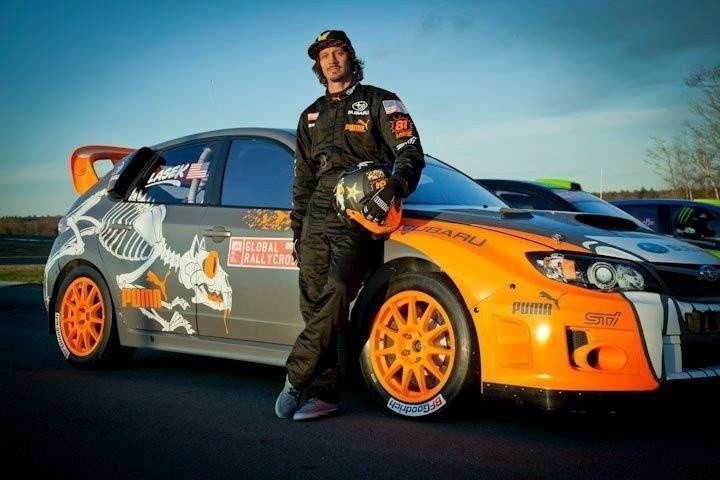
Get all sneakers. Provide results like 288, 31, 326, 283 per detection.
292, 395, 340, 420
274, 372, 304, 420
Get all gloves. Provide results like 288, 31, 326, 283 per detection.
291, 227, 306, 270
360, 185, 395, 225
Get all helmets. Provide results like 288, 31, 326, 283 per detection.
331, 159, 404, 242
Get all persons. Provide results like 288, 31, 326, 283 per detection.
272, 28, 426, 423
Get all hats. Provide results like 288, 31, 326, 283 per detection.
307, 28, 352, 61
